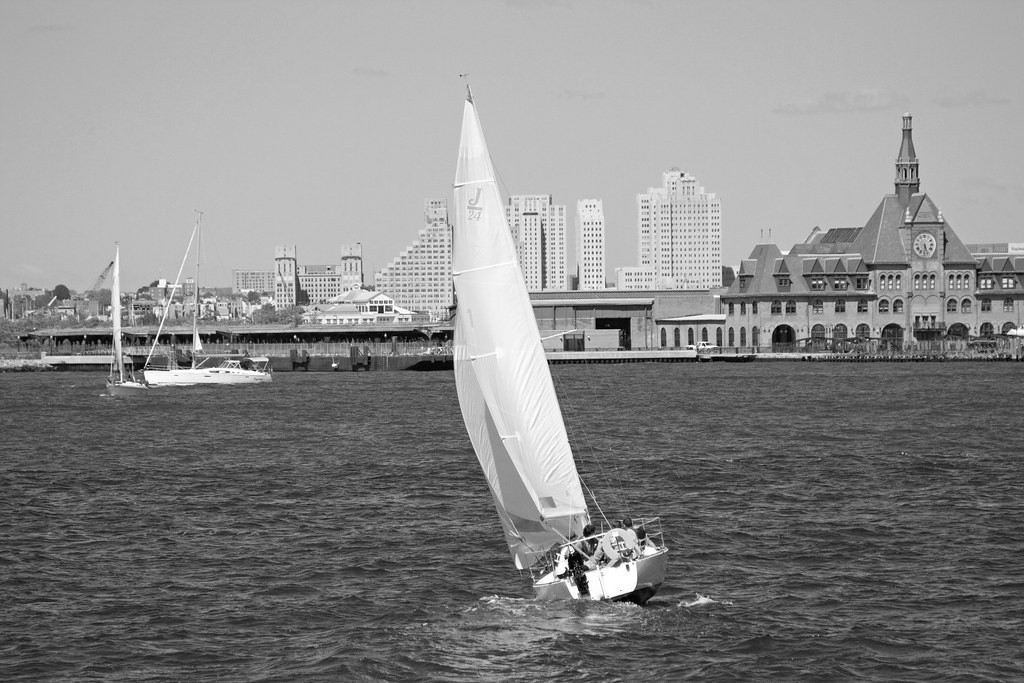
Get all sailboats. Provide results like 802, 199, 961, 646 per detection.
104, 244, 148, 389
448, 73, 667, 603
138, 211, 273, 388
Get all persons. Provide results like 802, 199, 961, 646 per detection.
574, 524, 603, 562
611, 518, 646, 561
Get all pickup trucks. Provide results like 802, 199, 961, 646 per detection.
686, 342, 719, 351
